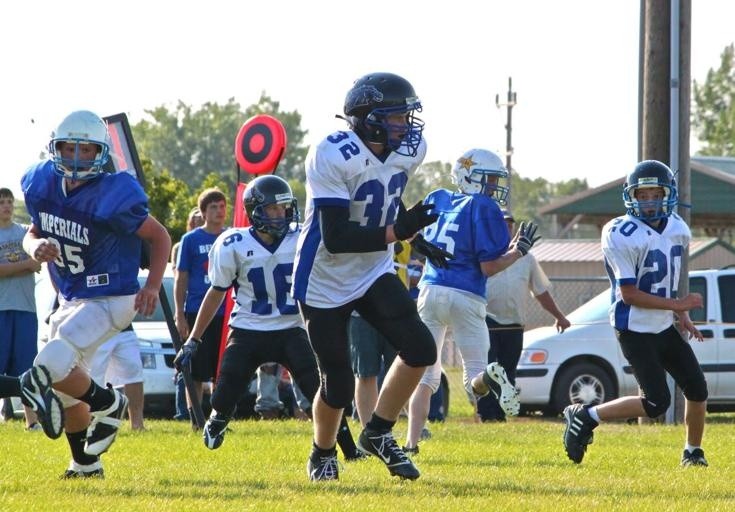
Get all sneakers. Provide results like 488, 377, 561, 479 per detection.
484, 361, 521, 416
203, 404, 238, 449
18, 366, 65, 439
308, 448, 342, 480
84, 383, 130, 456
61, 461, 103, 477
357, 420, 421, 479
681, 449, 708, 467
561, 403, 599, 464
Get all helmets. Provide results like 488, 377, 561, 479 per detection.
243, 174, 300, 235
500, 208, 515, 221
46, 111, 112, 183
623, 160, 679, 220
450, 148, 510, 205
345, 76, 424, 157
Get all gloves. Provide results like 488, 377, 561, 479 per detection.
174, 335, 202, 370
514, 221, 541, 255
394, 201, 438, 240
410, 234, 456, 271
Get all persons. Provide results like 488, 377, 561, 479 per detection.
171, 189, 231, 429
89, 322, 144, 432
290, 71, 439, 482
562, 160, 709, 465
403, 148, 542, 455
1, 188, 42, 432
345, 211, 445, 439
480, 207, 571, 424
231, 362, 308, 420
173, 174, 369, 461
22, 109, 171, 479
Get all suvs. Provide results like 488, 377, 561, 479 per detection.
511, 268, 735, 419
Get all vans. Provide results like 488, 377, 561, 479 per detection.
34, 264, 182, 415
